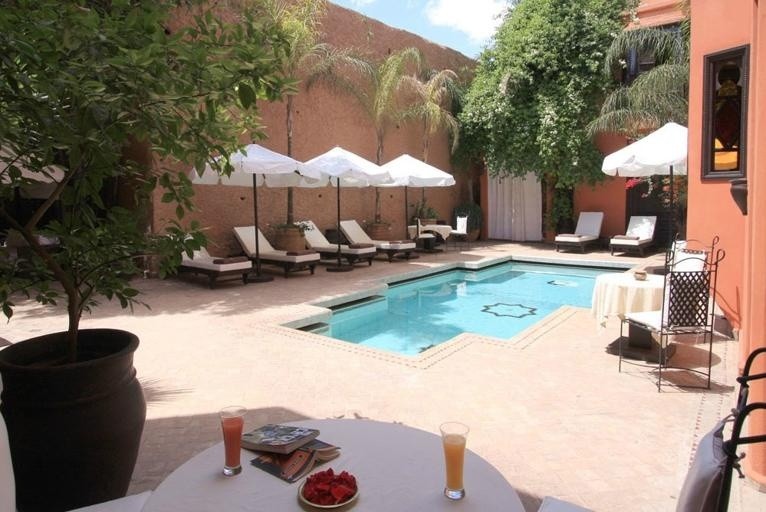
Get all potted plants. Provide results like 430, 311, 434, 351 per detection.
0, 0, 307, 512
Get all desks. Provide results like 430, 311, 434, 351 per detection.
591, 272, 675, 366
408, 225, 452, 253
144, 416, 526, 512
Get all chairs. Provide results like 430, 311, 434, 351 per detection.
166, 233, 253, 289
619, 249, 725, 392
0, 374, 152, 512
667, 232, 720, 281
537, 347, 766, 512
610, 216, 657, 257
555, 212, 604, 253
293, 220, 376, 266
446, 215, 469, 249
336, 220, 416, 264
415, 218, 435, 253
232, 226, 320, 278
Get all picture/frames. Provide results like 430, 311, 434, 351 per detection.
702, 44, 750, 181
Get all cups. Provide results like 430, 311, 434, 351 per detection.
219, 406, 247, 477
440, 423, 470, 499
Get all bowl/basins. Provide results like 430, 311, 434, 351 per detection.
634, 272, 647, 280
299, 473, 359, 510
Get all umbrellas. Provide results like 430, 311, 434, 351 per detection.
601, 122, 688, 269
300, 144, 395, 266
378, 154, 456, 255
189, 141, 303, 276
0, 139, 69, 200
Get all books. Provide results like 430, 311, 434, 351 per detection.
250, 439, 341, 484
240, 425, 320, 455
